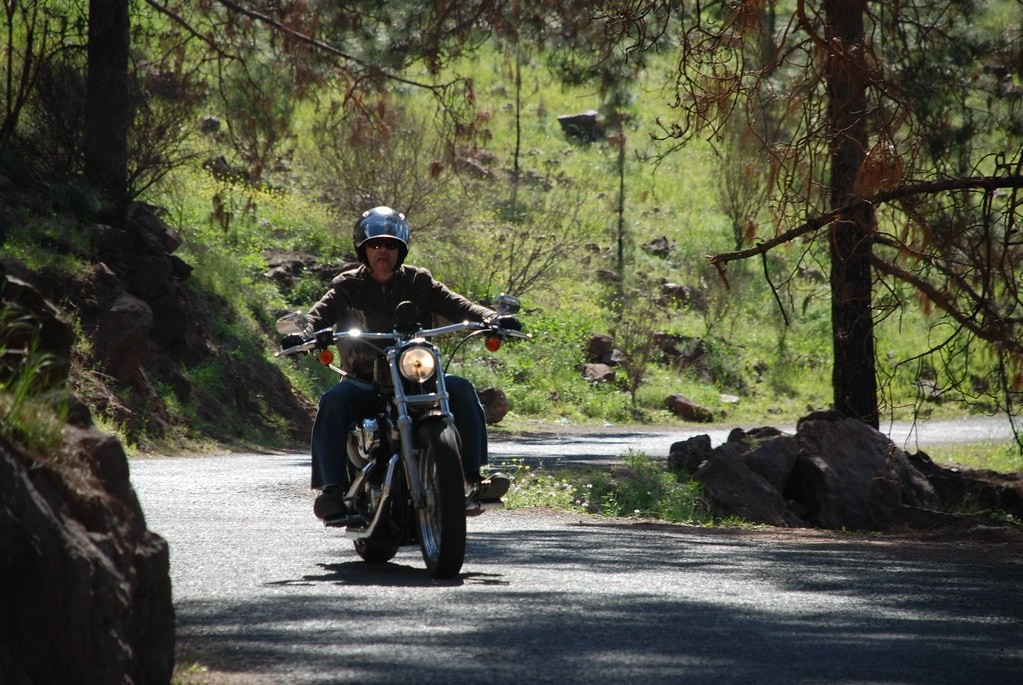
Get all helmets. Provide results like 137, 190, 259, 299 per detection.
352, 206, 411, 263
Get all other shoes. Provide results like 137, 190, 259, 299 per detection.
314, 483, 351, 528
466, 471, 510, 517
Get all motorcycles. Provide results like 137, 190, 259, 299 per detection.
275, 291, 532, 582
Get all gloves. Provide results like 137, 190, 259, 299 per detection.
280, 332, 308, 360
491, 315, 522, 340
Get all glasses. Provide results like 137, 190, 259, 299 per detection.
362, 238, 404, 250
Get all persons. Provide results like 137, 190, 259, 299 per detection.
281, 207, 523, 520
